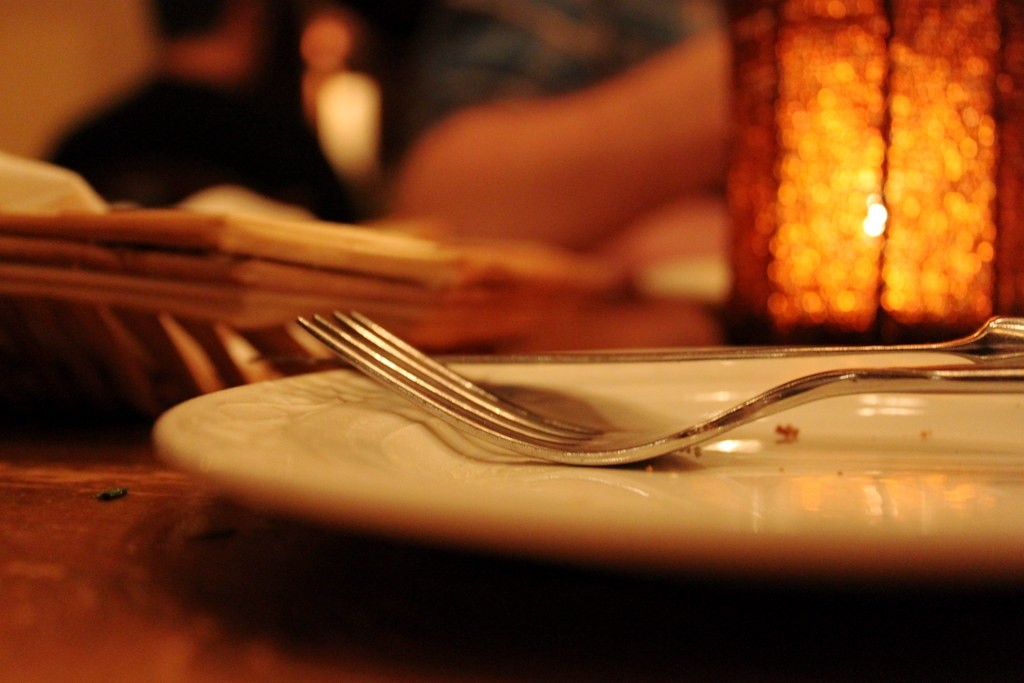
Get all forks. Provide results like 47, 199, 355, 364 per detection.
294, 306, 1024, 469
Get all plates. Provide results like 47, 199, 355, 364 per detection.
151, 348, 1024, 577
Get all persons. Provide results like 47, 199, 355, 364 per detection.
36, 1, 379, 231
380, 1, 734, 261
